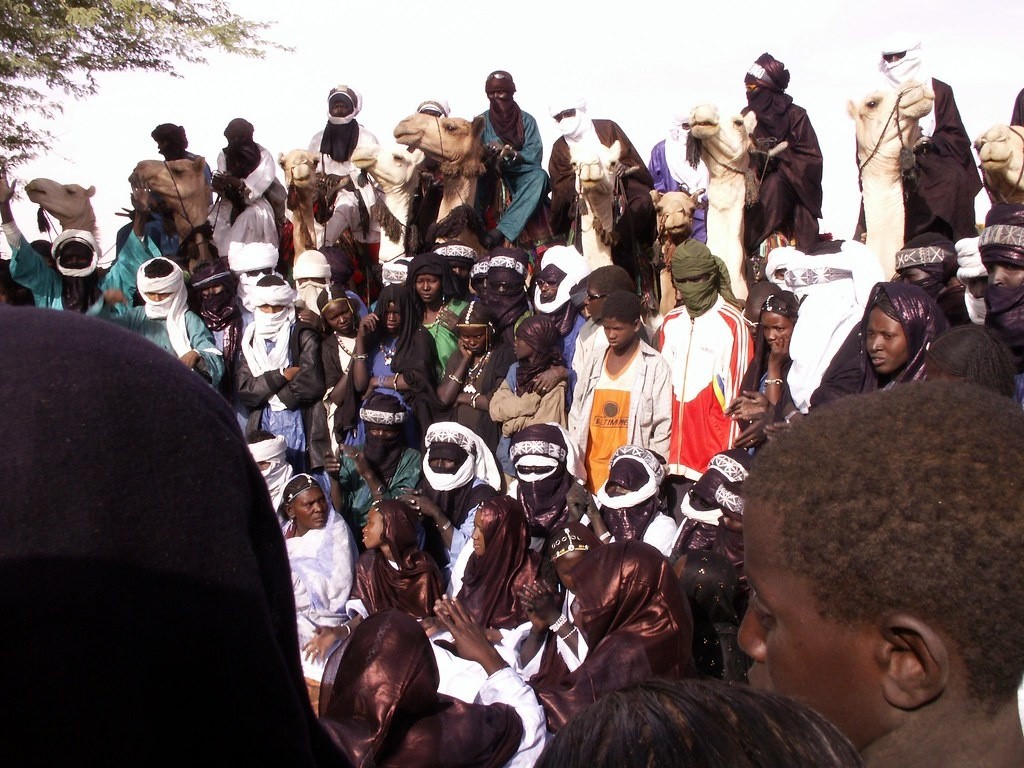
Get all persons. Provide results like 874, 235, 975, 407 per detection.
0, 34, 1024, 768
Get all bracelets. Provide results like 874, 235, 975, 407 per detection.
353, 353, 369, 359
599, 531, 610, 541
471, 395, 474, 406
344, 625, 350, 634
193, 349, 200, 354
449, 374, 462, 383
394, 373, 399, 391
472, 393, 480, 408
378, 376, 386, 388
549, 613, 567, 632
443, 520, 451, 530
513, 151, 517, 161
562, 628, 576, 641
764, 379, 783, 385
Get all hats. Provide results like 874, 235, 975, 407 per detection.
414, 92, 451, 118
293, 250, 332, 280
247, 395, 752, 768
151, 123, 188, 149
51, 229, 804, 393
223, 118, 255, 137
859, 203, 1023, 397
328, 85, 358, 110
548, 93, 590, 140
744, 52, 790, 93
485, 71, 516, 92
879, 30, 924, 82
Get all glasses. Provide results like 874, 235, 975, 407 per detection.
489, 280, 515, 291
586, 291, 607, 301
534, 278, 559, 288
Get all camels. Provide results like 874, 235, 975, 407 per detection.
571, 140, 620, 271
279, 149, 318, 254
355, 146, 421, 262
653, 190, 699, 315
687, 105, 754, 302
979, 126, 1024, 191
846, 79, 934, 269
24, 177, 102, 257
395, 115, 546, 262
130, 157, 294, 272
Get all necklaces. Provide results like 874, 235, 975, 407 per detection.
463, 351, 490, 394
379, 343, 395, 365
333, 331, 352, 356
427, 307, 442, 336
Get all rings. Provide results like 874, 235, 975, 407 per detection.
354, 454, 358, 456
316, 649, 320, 653
543, 388, 546, 389
